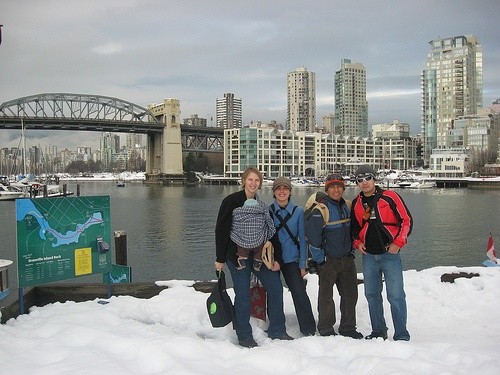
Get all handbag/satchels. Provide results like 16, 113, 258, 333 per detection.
249, 274, 267, 322
206, 270, 234, 328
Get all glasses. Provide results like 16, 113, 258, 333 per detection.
356, 176, 373, 183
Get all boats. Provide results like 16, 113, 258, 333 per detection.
0, 185, 24, 199
262, 178, 437, 189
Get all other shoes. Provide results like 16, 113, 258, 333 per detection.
339, 327, 363, 339
393, 329, 410, 341
318, 327, 338, 336
365, 331, 388, 341
305, 331, 315, 336
239, 338, 258, 348
270, 331, 294, 340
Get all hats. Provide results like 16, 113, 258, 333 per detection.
273, 177, 292, 191
355, 164, 377, 181
325, 174, 345, 192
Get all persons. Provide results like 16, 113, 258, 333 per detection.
215, 167, 294, 347
236, 199, 263, 272
349, 163, 413, 340
263, 177, 316, 337
305, 174, 364, 339
